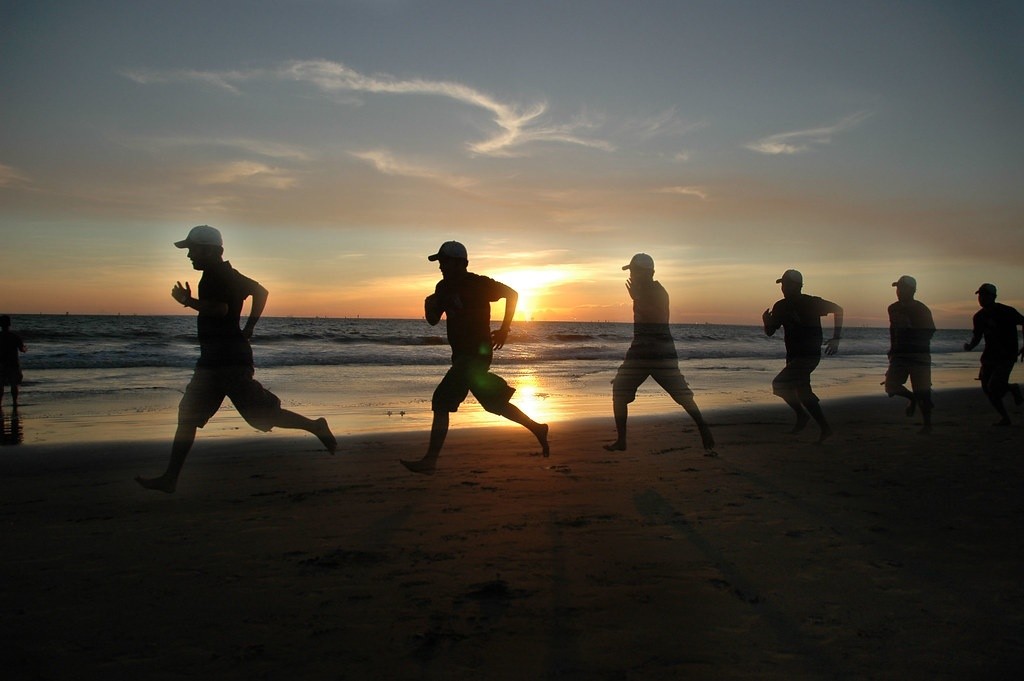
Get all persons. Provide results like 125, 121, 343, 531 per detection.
0, 315, 28, 430
963, 284, 1024, 429
763, 270, 842, 445
135, 224, 337, 493
398, 239, 550, 474
884, 274, 937, 435
603, 254, 715, 452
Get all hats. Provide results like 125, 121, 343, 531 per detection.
975, 282, 997, 294
776, 269, 803, 286
173, 224, 224, 248
427, 239, 468, 261
621, 251, 655, 271
892, 275, 917, 289
